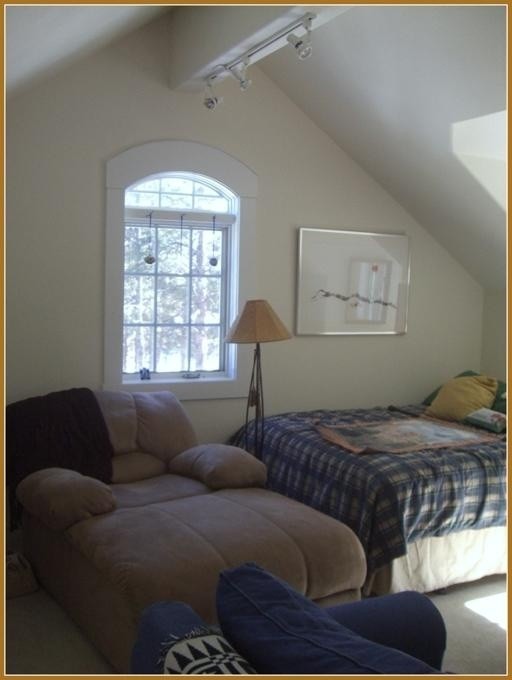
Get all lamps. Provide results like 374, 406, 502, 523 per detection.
221, 298, 292, 461
198, 11, 318, 111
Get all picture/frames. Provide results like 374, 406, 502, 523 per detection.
294, 228, 411, 336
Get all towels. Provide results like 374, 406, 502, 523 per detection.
6, 387, 111, 490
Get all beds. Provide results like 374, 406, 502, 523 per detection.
4, 384, 368, 675
221, 403, 508, 596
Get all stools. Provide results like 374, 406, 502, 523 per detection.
56, 488, 369, 675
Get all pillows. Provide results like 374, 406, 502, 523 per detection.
423, 368, 506, 421
14, 467, 118, 533
214, 559, 445, 674
170, 444, 267, 486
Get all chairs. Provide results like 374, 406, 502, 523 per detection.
129, 590, 456, 674
